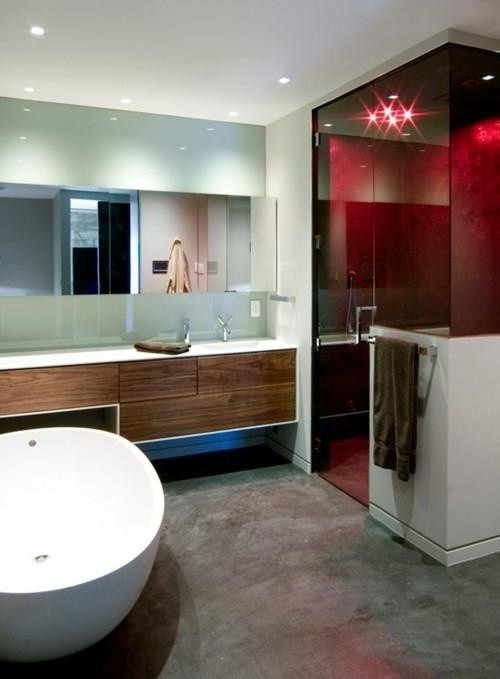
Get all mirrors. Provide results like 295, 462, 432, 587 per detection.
0, 181, 275, 297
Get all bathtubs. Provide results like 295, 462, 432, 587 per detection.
318, 325, 450, 347
0, 427, 165, 665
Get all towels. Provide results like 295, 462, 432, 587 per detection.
371, 337, 420, 483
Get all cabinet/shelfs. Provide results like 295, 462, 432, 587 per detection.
118, 349, 296, 442
0, 362, 121, 416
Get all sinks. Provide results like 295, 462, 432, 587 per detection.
190, 341, 260, 354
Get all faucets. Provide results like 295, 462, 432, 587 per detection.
354, 305, 376, 344
182, 317, 192, 347
216, 315, 232, 340
